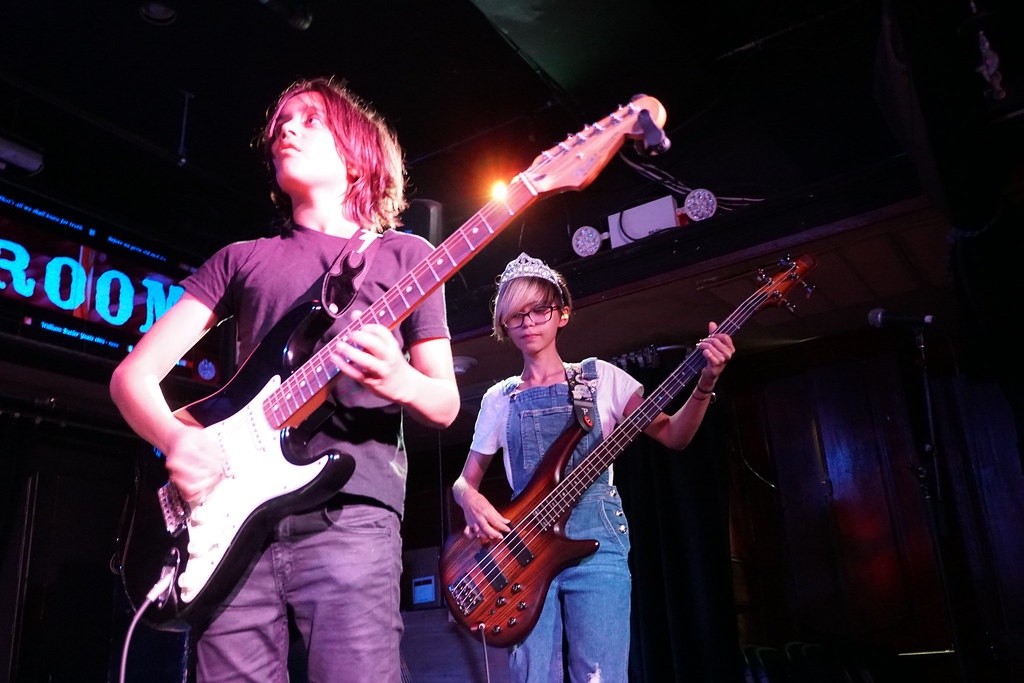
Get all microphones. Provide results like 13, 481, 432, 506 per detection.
867, 307, 935, 326
259, 0, 315, 32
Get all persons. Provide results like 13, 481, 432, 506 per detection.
453, 252, 736, 683
107, 76, 462, 683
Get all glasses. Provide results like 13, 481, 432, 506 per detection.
503, 305, 562, 329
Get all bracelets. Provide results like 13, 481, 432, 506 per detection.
691, 382, 714, 401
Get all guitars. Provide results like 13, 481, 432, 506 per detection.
439, 250, 822, 649
119, 93, 674, 634
